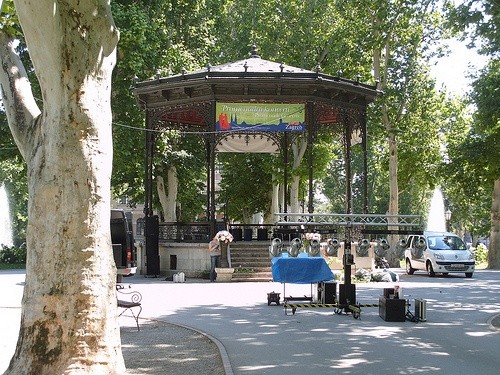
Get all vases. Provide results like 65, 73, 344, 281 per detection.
214, 267, 235, 282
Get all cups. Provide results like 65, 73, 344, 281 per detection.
301, 253, 307, 257
282, 253, 288, 258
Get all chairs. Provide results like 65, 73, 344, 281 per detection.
116, 285, 142, 332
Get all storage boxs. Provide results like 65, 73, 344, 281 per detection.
379, 297, 406, 321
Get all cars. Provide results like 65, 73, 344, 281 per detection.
404, 233, 476, 278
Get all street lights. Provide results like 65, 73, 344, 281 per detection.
444, 207, 452, 232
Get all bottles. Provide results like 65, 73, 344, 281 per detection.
394, 281, 400, 299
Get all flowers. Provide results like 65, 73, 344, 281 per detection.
215, 230, 233, 268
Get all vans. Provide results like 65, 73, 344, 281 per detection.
107, 209, 137, 284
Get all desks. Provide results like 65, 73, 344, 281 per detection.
271, 252, 334, 304
